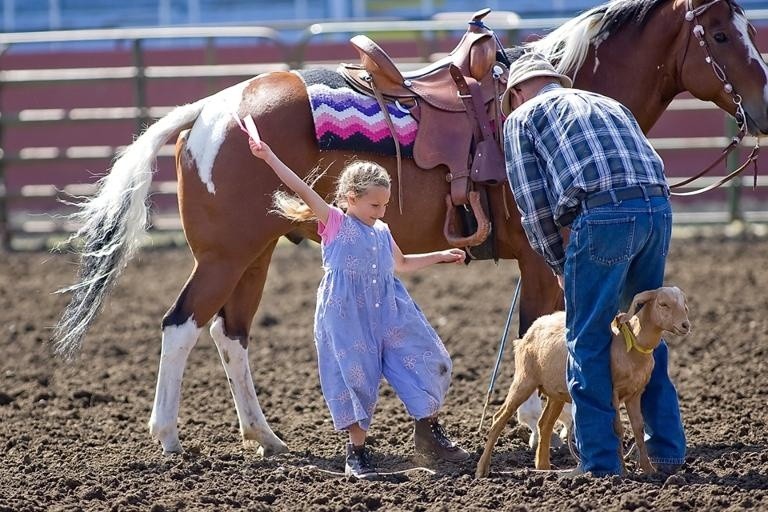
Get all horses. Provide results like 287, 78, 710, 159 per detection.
40, 0, 768, 458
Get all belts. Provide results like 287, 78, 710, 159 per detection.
556, 184, 672, 226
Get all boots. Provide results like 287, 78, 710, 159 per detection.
344, 443, 380, 482
412, 414, 472, 462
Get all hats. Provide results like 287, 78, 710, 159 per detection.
500, 51, 574, 119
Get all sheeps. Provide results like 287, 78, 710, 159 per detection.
475, 285, 691, 478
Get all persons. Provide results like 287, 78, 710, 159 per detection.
493, 51, 689, 483
246, 135, 470, 480
